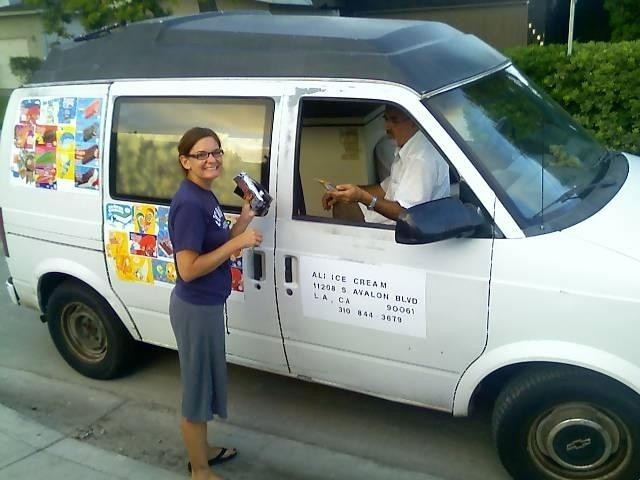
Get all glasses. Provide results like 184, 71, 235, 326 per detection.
382, 113, 411, 125
184, 149, 224, 160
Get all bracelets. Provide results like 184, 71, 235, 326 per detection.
366, 193, 378, 211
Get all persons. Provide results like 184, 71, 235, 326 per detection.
322, 102, 452, 229
167, 127, 263, 476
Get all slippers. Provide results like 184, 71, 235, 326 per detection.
188, 445, 237, 474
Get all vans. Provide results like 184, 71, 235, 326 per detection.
1, 10, 639, 479
